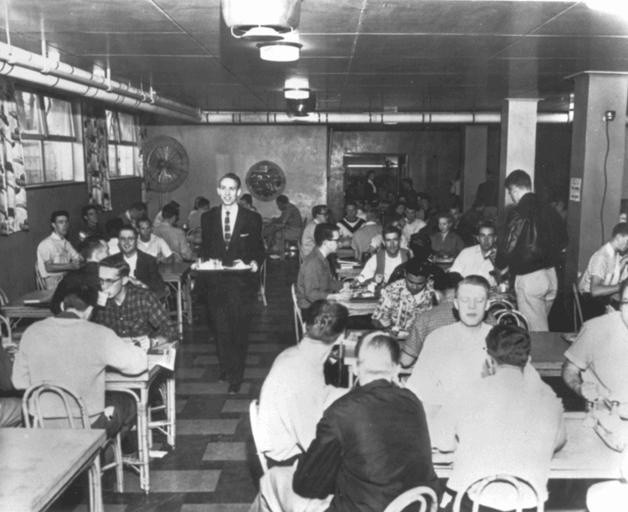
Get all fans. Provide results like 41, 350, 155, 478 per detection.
140, 135, 189, 212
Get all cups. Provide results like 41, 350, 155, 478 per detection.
97, 292, 109, 308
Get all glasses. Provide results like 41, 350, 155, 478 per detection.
97, 278, 121, 285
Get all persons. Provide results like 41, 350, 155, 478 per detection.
292, 335, 444, 512
198, 169, 628, 417
255, 299, 335, 512
2, 169, 206, 491
435, 324, 569, 512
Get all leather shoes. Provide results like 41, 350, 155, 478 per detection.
217, 371, 229, 383
227, 383, 240, 395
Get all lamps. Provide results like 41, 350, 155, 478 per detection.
283, 71, 312, 100
219, 1, 302, 63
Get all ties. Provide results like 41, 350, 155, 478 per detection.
225, 211, 231, 250
